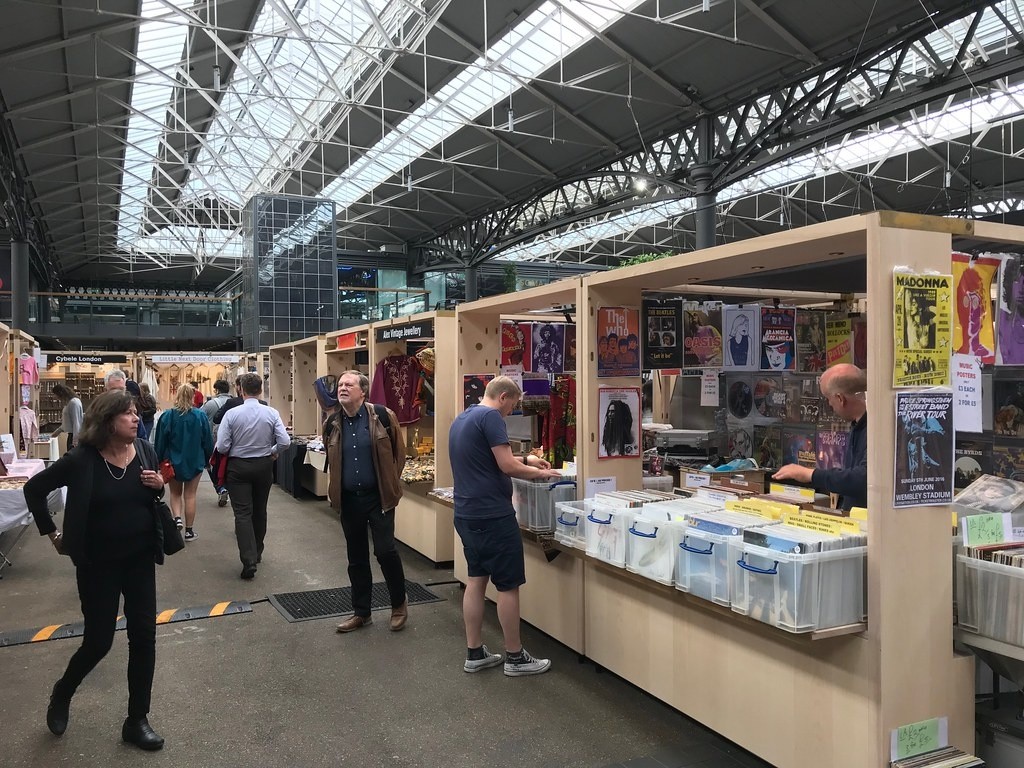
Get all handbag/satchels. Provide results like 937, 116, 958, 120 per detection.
159, 458, 175, 484
134, 437, 185, 557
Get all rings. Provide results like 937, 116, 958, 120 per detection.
144, 475, 147, 479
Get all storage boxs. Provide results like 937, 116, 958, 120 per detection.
512, 429, 1024, 648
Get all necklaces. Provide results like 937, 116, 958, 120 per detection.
104, 442, 128, 479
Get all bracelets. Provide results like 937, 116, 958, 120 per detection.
524, 457, 527, 464
52, 533, 61, 546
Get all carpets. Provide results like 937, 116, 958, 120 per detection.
266, 577, 448, 623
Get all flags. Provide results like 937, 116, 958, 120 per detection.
805, 352, 826, 371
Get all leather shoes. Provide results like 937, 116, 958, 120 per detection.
389, 594, 408, 631
121, 717, 165, 749
336, 613, 373, 632
47, 681, 72, 734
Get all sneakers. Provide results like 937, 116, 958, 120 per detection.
503, 648, 552, 676
464, 646, 503, 673
185, 530, 199, 542
173, 518, 184, 532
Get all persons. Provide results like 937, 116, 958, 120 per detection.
534, 324, 561, 373
771, 363, 868, 511
686, 310, 723, 366
508, 329, 526, 372
122, 369, 146, 418
760, 429, 777, 466
201, 380, 234, 506
568, 332, 576, 360
955, 381, 1024, 513
602, 402, 635, 456
190, 381, 203, 408
449, 375, 563, 677
154, 384, 214, 542
213, 374, 267, 424
51, 384, 83, 452
138, 382, 157, 441
217, 372, 290, 579
23, 389, 166, 746
322, 370, 409, 632
104, 368, 147, 440
805, 312, 823, 354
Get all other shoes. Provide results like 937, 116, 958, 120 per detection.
241, 564, 257, 579
218, 489, 228, 507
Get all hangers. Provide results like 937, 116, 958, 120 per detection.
151, 363, 225, 371
20, 405, 27, 409
388, 344, 404, 357
20, 347, 29, 357
